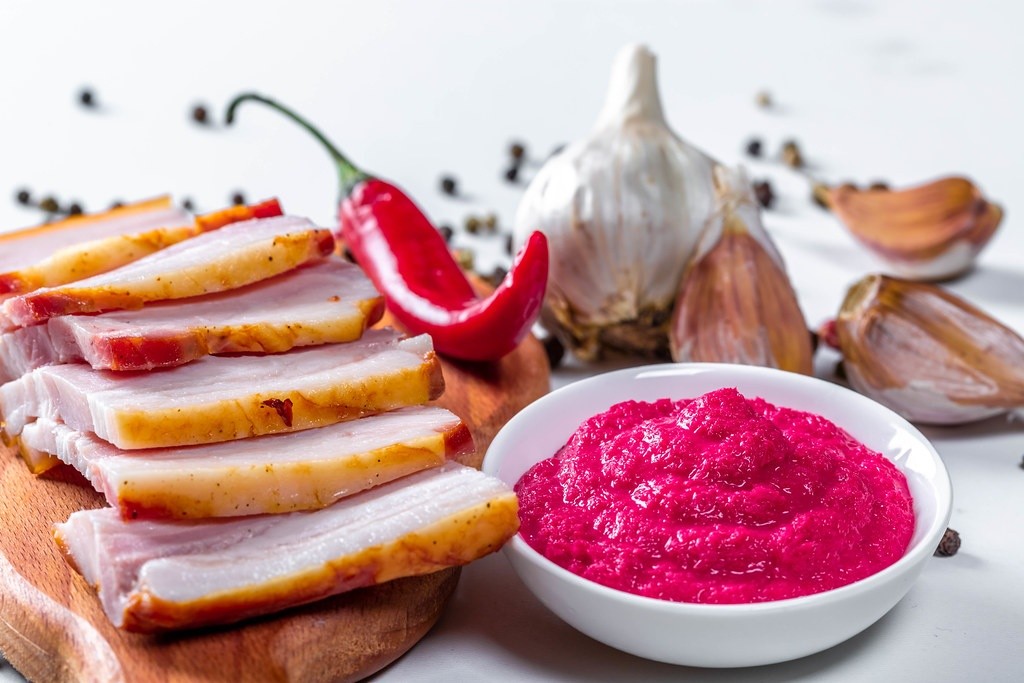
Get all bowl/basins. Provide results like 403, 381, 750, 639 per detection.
479, 361, 954, 666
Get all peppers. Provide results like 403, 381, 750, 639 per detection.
226, 94, 548, 364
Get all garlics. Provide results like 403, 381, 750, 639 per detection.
517, 42, 1024, 426
